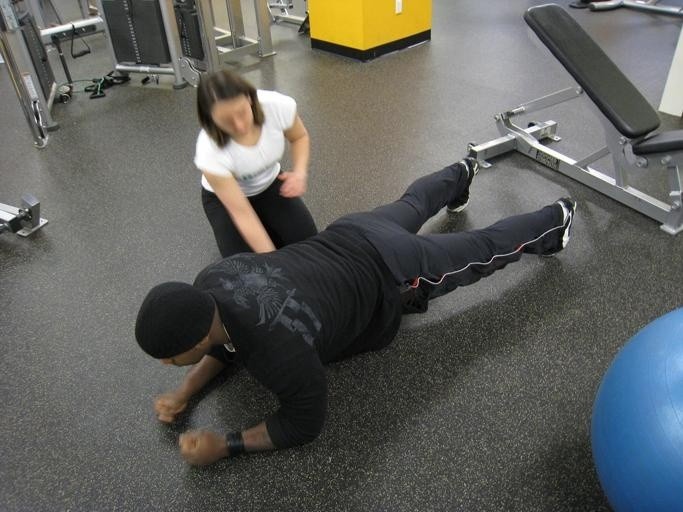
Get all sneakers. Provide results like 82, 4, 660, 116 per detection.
542, 198, 578, 258
447, 156, 481, 214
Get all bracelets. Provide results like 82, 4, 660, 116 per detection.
223, 431, 247, 456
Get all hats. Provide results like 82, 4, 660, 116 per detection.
135, 283, 216, 359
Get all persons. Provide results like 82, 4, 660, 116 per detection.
190, 69, 318, 259
132, 155, 577, 468
